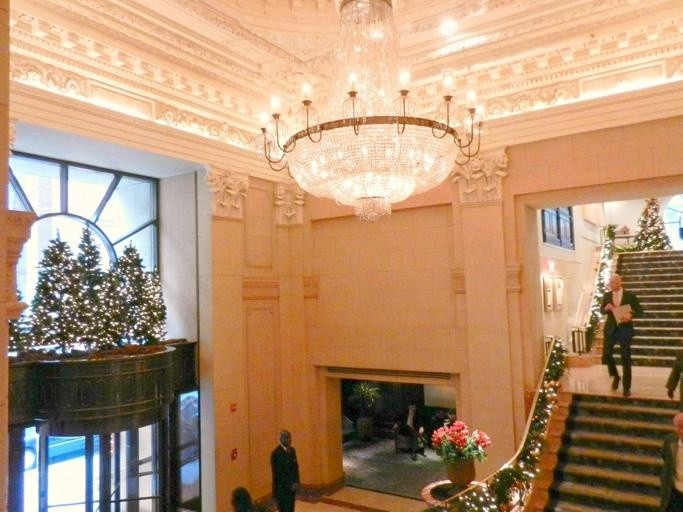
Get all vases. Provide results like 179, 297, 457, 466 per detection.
446, 456, 475, 485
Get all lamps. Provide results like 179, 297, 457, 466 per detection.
252, 0, 485, 227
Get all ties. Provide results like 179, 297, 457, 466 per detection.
614, 294, 619, 306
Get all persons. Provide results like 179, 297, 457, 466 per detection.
599, 272, 644, 396
658, 411, 683, 511
666, 350, 683, 410
270, 428, 301, 512
230, 486, 251, 511
390, 400, 427, 461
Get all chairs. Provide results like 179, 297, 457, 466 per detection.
393, 423, 425, 456
341, 415, 355, 449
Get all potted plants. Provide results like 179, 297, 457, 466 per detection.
347, 382, 382, 442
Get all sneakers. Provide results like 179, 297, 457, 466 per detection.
624, 389, 631, 395
612, 376, 620, 389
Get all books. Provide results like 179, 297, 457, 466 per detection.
612, 304, 633, 325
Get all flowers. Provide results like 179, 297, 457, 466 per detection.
431, 421, 493, 462
